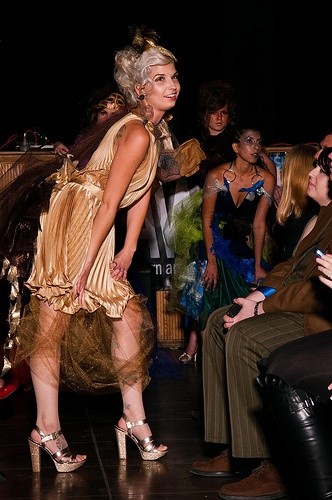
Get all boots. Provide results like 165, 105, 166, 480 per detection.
254, 374, 332, 500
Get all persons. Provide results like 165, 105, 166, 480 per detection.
259, 152, 277, 179
189, 134, 332, 500
164, 120, 276, 336
11, 25, 181, 472
53, 93, 121, 157
176, 80, 239, 363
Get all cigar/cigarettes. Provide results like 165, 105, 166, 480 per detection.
317, 250, 324, 256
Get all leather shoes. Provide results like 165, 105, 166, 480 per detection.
190, 448, 254, 477
0, 378, 19, 399
218, 461, 287, 500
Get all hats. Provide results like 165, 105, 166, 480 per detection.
94, 92, 126, 113
313, 147, 332, 177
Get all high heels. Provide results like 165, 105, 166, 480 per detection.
178, 352, 197, 363
114, 414, 168, 461
28, 426, 87, 473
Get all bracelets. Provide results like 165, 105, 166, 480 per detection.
254, 301, 264, 315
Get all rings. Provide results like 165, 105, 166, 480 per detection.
113, 262, 117, 267
